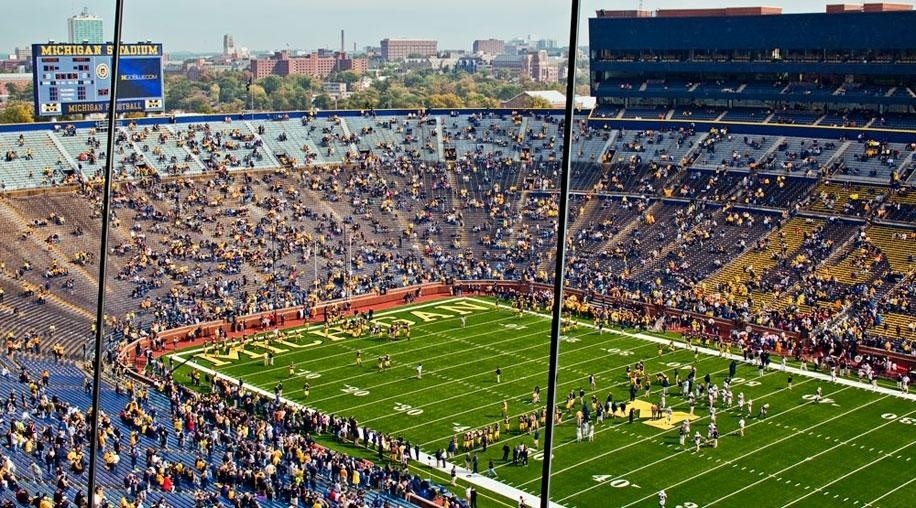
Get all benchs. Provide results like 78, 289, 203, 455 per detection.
1, 106, 544, 349
0, 351, 454, 508
540, 56, 916, 363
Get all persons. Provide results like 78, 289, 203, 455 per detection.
1, 49, 916, 507
